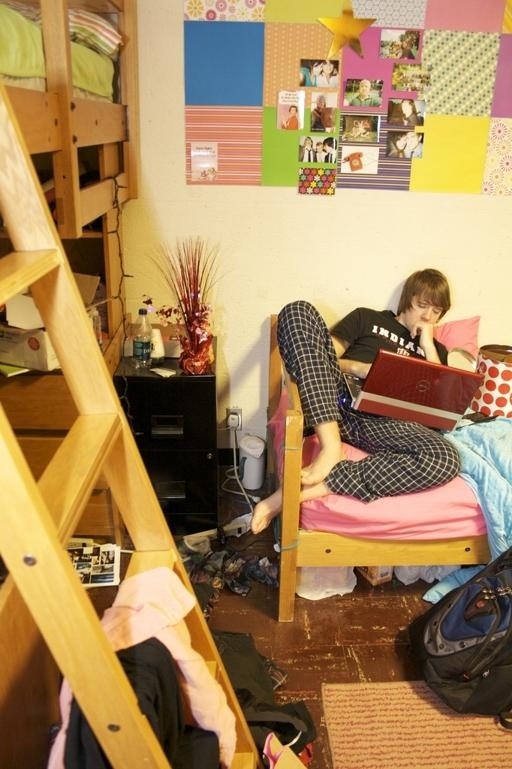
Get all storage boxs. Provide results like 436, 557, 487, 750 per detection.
1, 272, 99, 372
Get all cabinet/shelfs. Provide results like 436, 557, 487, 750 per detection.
0, 145, 124, 554
116, 335, 217, 533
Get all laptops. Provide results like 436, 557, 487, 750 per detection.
341, 347, 486, 433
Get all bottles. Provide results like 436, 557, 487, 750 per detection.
133, 308, 152, 372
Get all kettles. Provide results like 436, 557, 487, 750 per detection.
238, 433, 265, 490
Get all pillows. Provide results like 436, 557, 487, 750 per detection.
432, 318, 480, 360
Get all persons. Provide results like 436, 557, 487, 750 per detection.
281, 105, 298, 130
319, 137, 337, 164
312, 95, 335, 134
383, 34, 418, 60
316, 59, 338, 88
400, 98, 421, 126
300, 59, 324, 86
300, 137, 316, 163
404, 131, 424, 158
251, 266, 461, 537
314, 141, 328, 163
386, 130, 408, 158
349, 80, 380, 107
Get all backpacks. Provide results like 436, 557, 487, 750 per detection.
408, 544, 512, 729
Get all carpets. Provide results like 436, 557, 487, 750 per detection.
319, 681, 511, 768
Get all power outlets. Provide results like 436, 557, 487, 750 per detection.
226, 407, 242, 432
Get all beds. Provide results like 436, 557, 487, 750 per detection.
0, 0, 134, 239
267, 315, 512, 624
